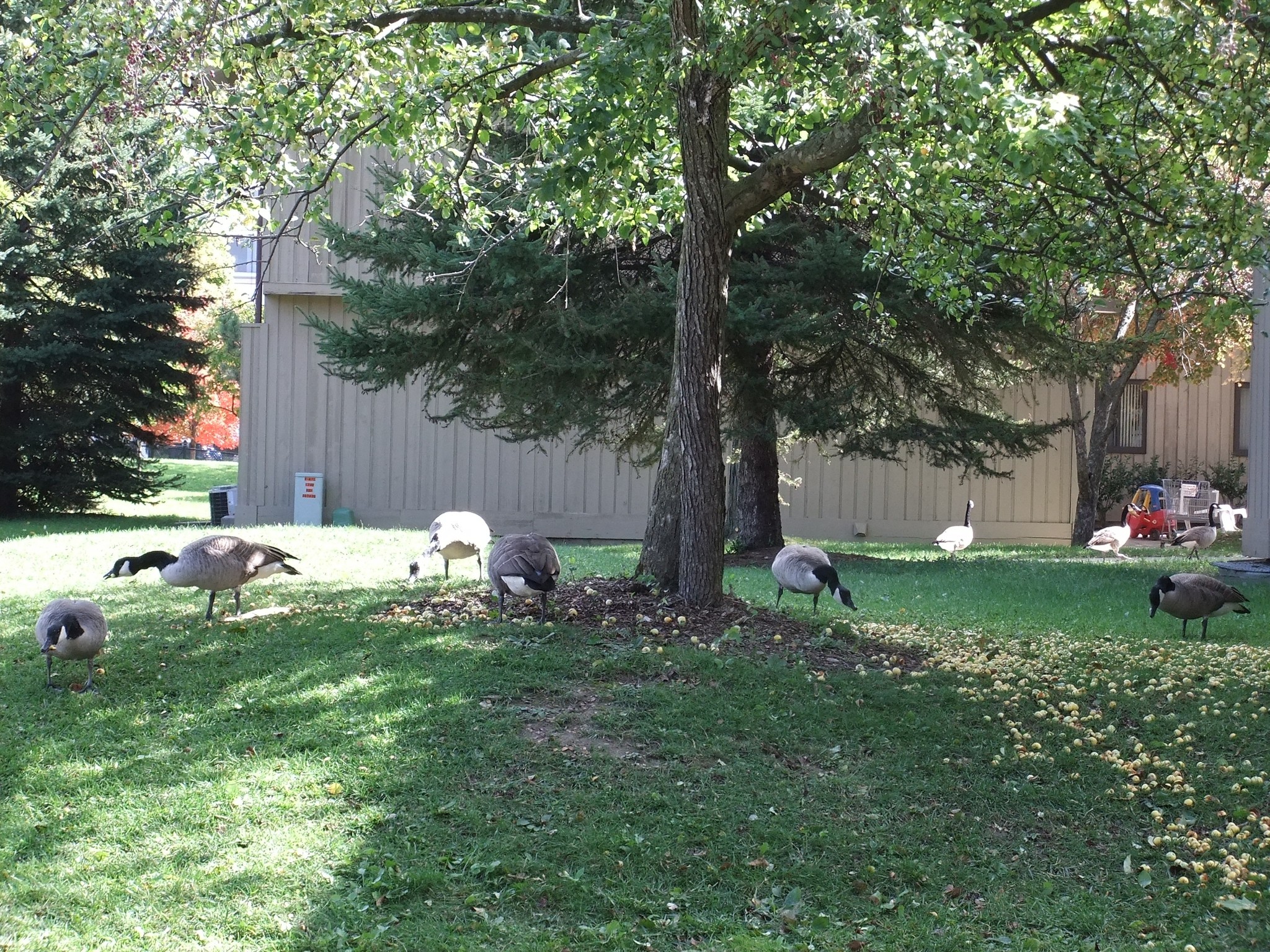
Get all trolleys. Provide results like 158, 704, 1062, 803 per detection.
1159, 479, 1220, 547
1120, 484, 1178, 540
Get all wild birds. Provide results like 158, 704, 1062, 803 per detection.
488, 533, 560, 625
931, 500, 974, 561
1149, 573, 1251, 642
409, 510, 495, 581
1081, 503, 1140, 561
103, 535, 303, 621
771, 545, 857, 612
1171, 503, 1222, 560
34, 599, 108, 693
